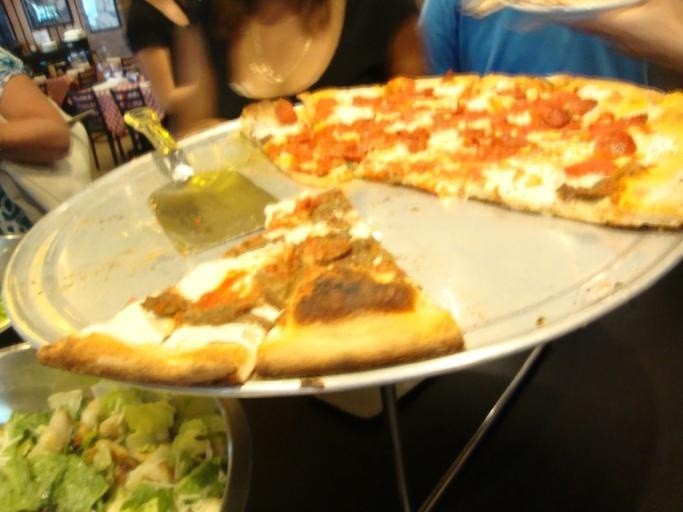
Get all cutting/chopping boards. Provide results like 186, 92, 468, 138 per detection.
3, 70, 683, 401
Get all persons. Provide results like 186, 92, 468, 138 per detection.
158, 1, 428, 138
409, 0, 681, 96
0, 47, 76, 228
120, 1, 209, 123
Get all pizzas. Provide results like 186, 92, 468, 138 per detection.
37, 189, 464, 386
238, 71, 683, 229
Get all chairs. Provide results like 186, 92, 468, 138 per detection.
39, 57, 167, 171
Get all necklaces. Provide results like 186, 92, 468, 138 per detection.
246, 9, 317, 85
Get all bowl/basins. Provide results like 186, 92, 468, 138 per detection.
0, 339, 254, 512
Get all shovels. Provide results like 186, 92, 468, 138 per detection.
124, 106, 279, 255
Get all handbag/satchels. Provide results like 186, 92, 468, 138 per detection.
3, 97, 91, 224
